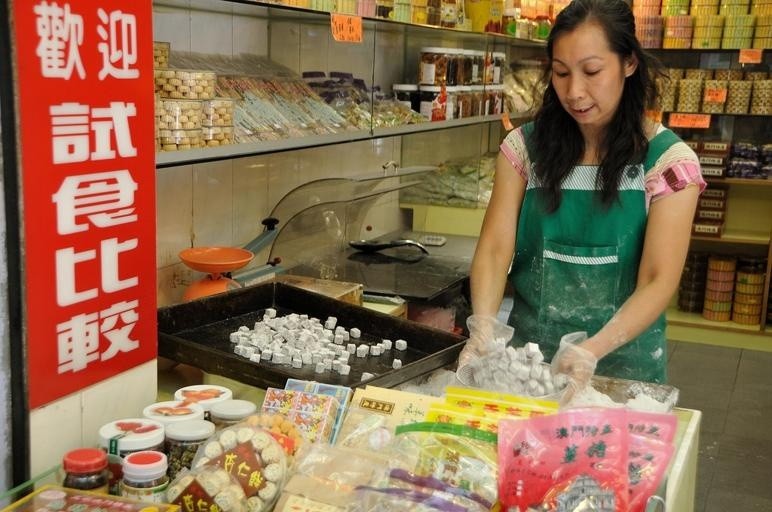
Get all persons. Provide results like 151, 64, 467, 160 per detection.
458, 1, 708, 409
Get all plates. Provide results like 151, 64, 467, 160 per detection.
180, 246, 255, 273
454, 356, 568, 406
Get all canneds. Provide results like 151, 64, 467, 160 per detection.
393, 46, 506, 123
62, 385, 257, 496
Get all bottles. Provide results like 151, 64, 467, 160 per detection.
502, 9, 550, 39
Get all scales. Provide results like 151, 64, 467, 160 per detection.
178, 246, 255, 304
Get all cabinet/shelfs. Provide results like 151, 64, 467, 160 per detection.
154, 1, 549, 309
501, 1, 772, 336
